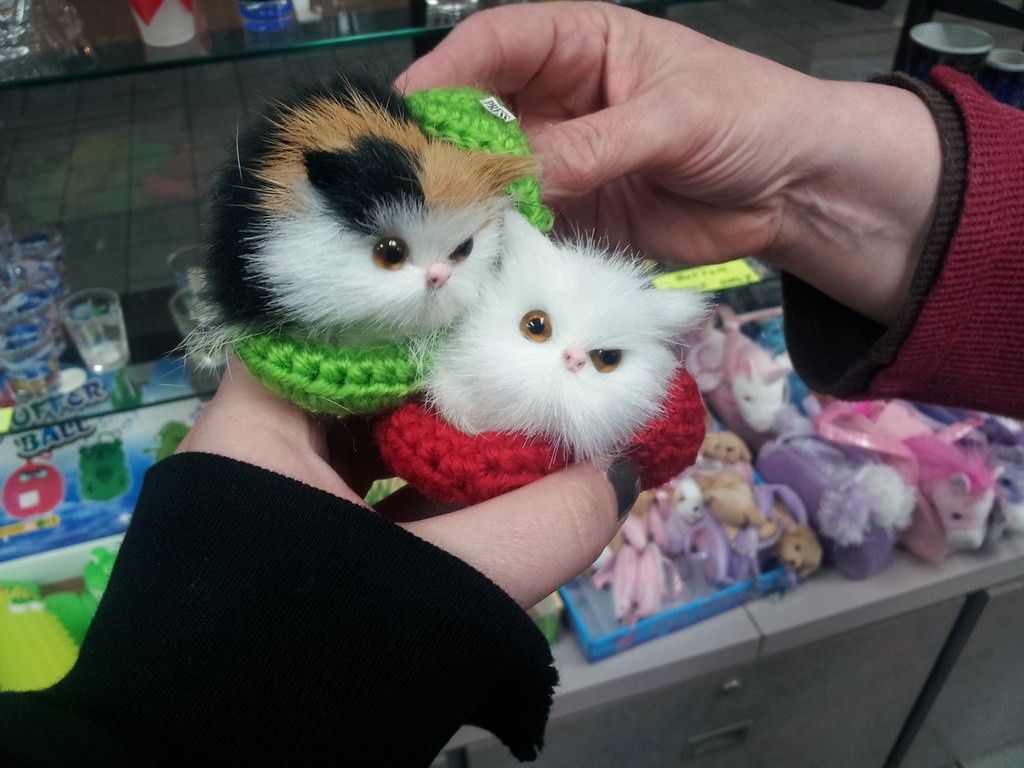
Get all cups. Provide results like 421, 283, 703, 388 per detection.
59, 286, 131, 375
908, 20, 996, 77
0, 222, 66, 398
169, 290, 220, 342
984, 48, 1024, 108
167, 244, 213, 289
188, 336, 231, 402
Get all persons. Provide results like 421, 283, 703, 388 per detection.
0, 335, 642, 767
388, 0, 1024, 421
